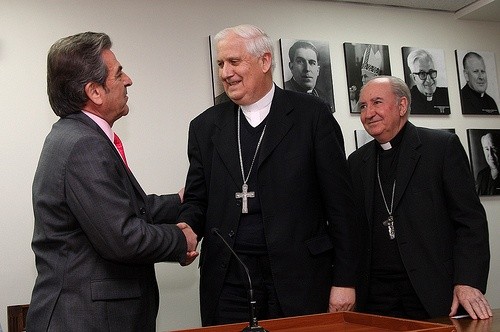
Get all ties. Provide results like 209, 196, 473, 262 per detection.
114, 132, 128, 171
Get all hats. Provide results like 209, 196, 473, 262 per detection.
361, 45, 382, 77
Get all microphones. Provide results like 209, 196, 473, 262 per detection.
210, 227, 269, 332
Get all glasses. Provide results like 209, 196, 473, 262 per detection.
413, 70, 437, 80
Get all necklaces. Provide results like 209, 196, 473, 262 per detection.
236, 105, 266, 213
377, 146, 396, 239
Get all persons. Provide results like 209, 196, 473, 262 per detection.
477, 130, 500, 196
24, 31, 197, 332
460, 52, 500, 115
175, 24, 347, 328
350, 44, 384, 112
348, 76, 492, 319
406, 49, 451, 115
284, 40, 335, 111
